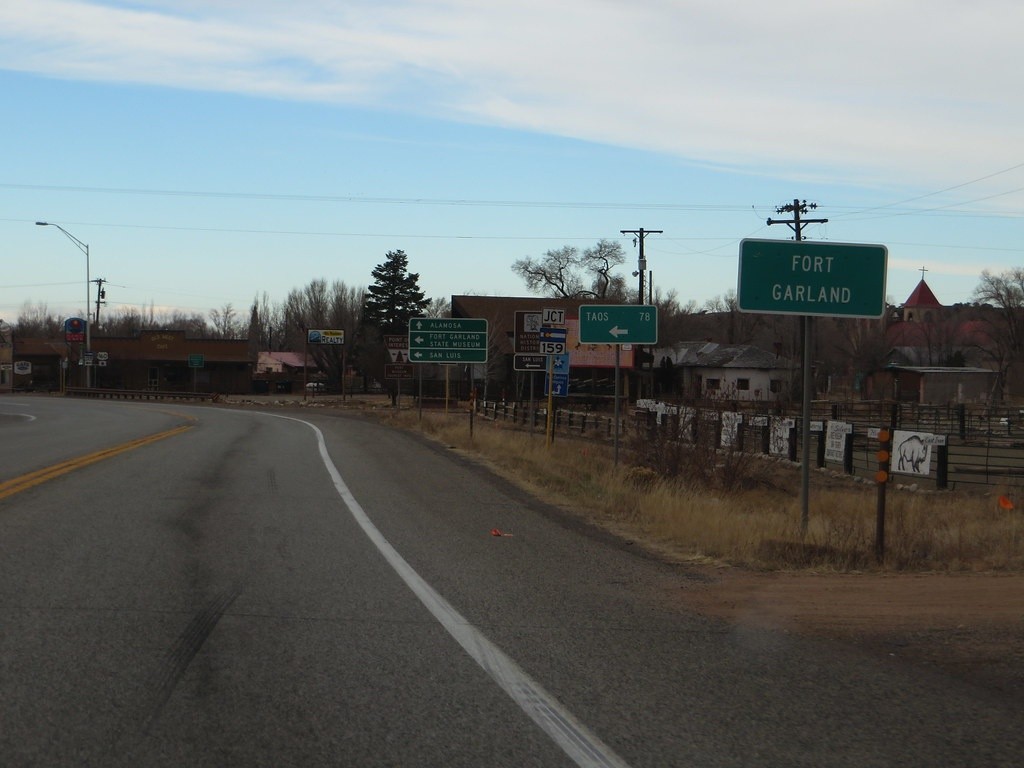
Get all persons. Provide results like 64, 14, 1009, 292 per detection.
388, 379, 398, 405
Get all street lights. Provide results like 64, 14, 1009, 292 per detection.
35, 221, 91, 389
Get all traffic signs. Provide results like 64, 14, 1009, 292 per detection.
579, 304, 659, 344
512, 351, 546, 371
544, 373, 569, 398
407, 317, 489, 364
